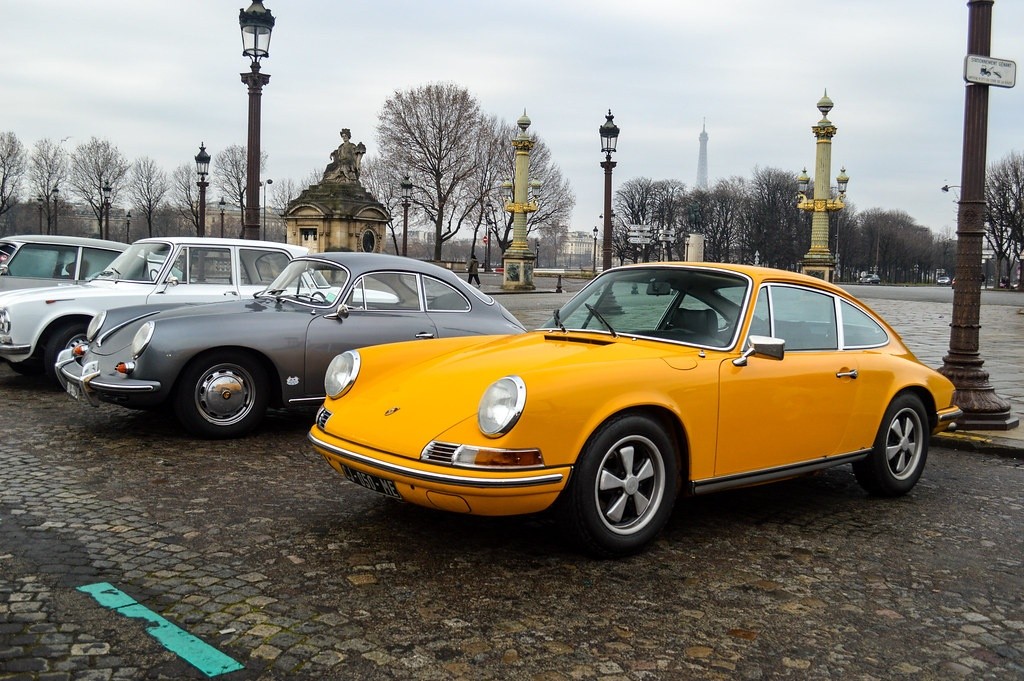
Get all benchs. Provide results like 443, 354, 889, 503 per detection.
533, 268, 565, 278
762, 319, 877, 347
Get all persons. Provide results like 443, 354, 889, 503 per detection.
468, 255, 481, 288
981, 272, 1010, 289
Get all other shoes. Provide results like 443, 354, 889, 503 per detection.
478, 284, 481, 288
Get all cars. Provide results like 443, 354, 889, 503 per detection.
307, 262, 962, 556
937, 277, 956, 289
55, 252, 529, 441
1, 234, 399, 389
860, 274, 881, 284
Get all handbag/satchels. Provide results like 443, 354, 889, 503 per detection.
469, 265, 473, 272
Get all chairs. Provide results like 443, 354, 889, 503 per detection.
53, 260, 89, 280
665, 308, 718, 339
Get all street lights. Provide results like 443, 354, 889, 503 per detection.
194, 142, 226, 238
400, 173, 413, 257
836, 217, 842, 281
593, 226, 598, 272
36, 179, 132, 244
536, 244, 540, 268
502, 108, 542, 290
263, 179, 273, 241
589, 109, 625, 316
796, 88, 849, 284
484, 219, 493, 272
239, 0, 275, 241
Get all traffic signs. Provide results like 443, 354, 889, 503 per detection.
628, 225, 650, 231
627, 238, 649, 244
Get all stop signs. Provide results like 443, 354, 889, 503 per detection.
483, 236, 487, 242
